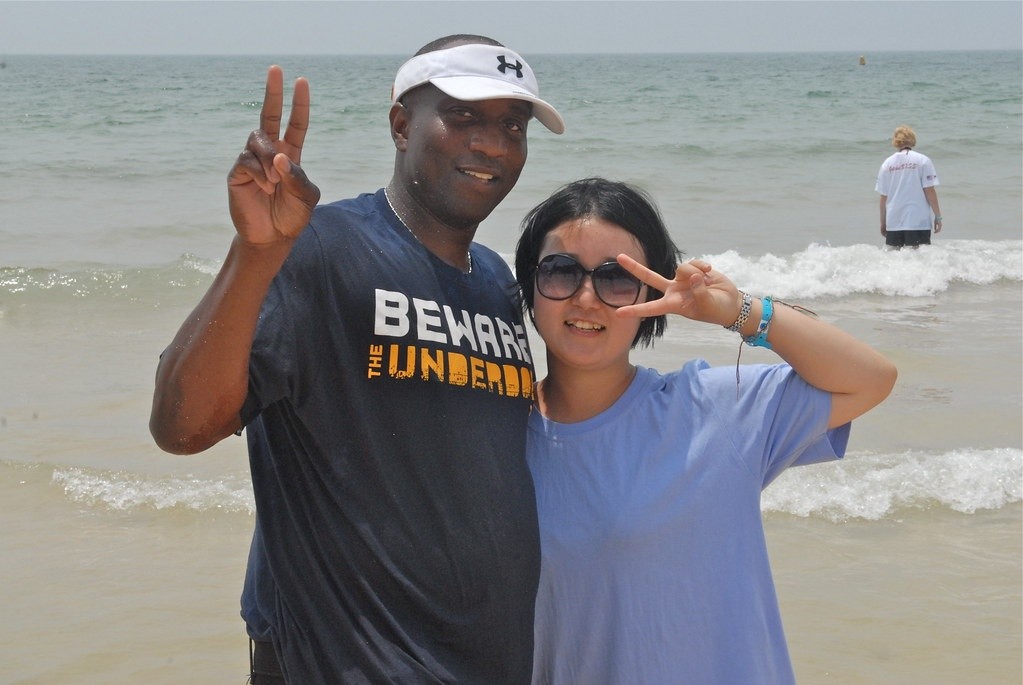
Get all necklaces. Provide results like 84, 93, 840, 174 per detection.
900, 147, 911, 155
384, 187, 472, 273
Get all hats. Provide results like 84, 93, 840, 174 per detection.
390, 44, 565, 135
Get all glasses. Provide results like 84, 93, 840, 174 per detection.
534, 254, 646, 308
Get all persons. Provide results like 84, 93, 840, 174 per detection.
149, 33, 566, 685
515, 176, 897, 685
875, 127, 942, 251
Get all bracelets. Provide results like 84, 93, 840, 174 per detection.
723, 291, 752, 332
935, 216, 942, 220
735, 296, 818, 395
